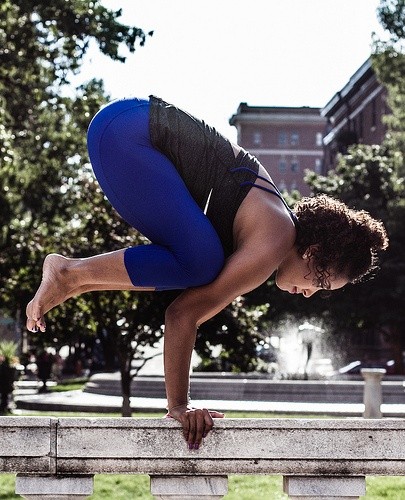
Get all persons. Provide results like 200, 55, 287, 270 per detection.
23, 91, 391, 452
